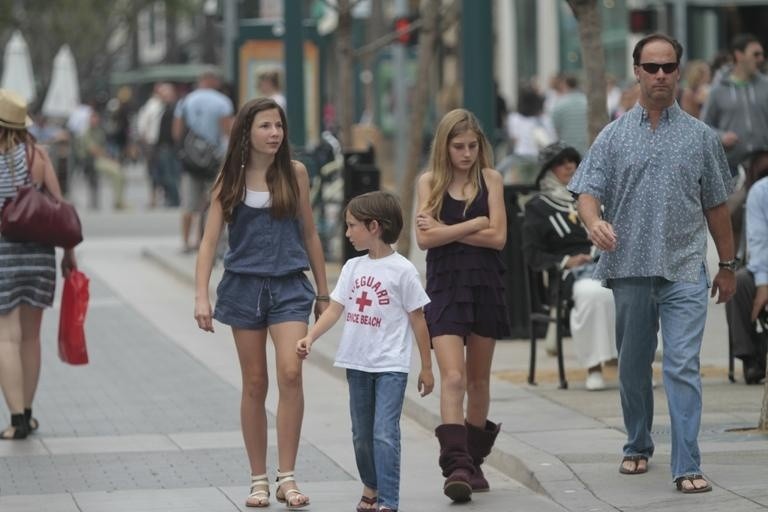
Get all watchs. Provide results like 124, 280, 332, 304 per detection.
716, 259, 737, 274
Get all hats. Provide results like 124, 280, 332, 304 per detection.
0, 88, 34, 130
532, 139, 581, 182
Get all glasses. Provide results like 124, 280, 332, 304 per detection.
637, 60, 680, 74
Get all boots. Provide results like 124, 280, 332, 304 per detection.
466, 419, 503, 492
434, 422, 473, 503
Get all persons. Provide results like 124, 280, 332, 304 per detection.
417, 108, 508, 504
248, 64, 288, 122
601, 73, 644, 123
321, 122, 380, 269
711, 54, 735, 90
296, 191, 433, 511
725, 175, 768, 383
0, 89, 78, 441
522, 139, 619, 390
696, 33, 768, 178
171, 63, 234, 253
17, 74, 177, 211
193, 99, 332, 510
676, 57, 712, 120
564, 35, 740, 495
728, 149, 767, 265
480, 66, 593, 174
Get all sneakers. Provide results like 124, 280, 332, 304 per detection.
586, 370, 605, 391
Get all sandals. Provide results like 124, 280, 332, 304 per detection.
22, 406, 39, 436
2, 413, 27, 441
274, 470, 311, 509
245, 472, 273, 508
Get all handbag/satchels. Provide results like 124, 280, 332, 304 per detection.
52, 265, 96, 366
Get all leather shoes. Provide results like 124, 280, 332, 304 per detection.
744, 361, 767, 386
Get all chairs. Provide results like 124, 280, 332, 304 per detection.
515, 214, 569, 391
725, 203, 746, 382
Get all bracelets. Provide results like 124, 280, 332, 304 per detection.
315, 296, 331, 302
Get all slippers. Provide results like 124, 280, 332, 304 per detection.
673, 472, 712, 493
357, 494, 378, 512
619, 448, 649, 475
